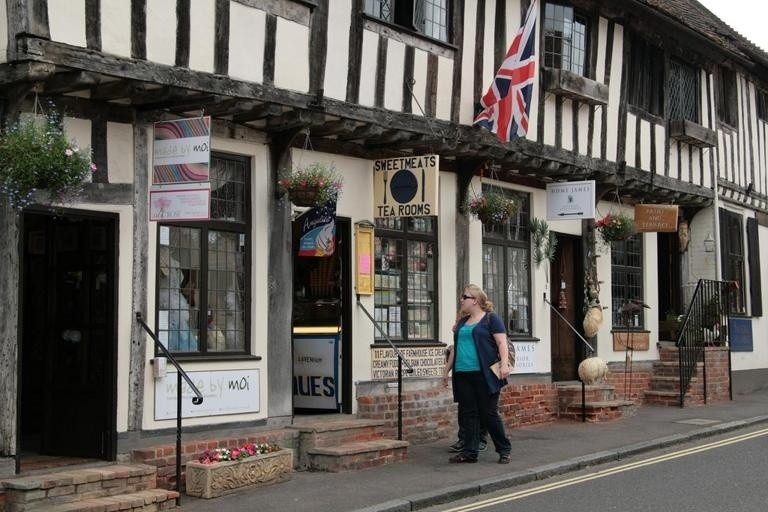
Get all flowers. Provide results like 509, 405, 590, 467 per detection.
466, 189, 515, 225
0, 104, 97, 213
282, 160, 343, 210
194, 442, 278, 464
593, 205, 636, 250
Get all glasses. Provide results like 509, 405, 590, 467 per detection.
458, 294, 478, 301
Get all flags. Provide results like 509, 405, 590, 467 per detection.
296, 189, 336, 257
471, 0, 540, 143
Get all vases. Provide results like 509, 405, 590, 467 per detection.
186, 448, 293, 498
611, 231, 631, 241
479, 211, 509, 225
288, 187, 321, 207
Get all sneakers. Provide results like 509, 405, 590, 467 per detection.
498, 451, 512, 465
448, 437, 488, 452
445, 453, 478, 465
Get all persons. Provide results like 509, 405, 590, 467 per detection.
444, 312, 488, 452
448, 283, 510, 464
701, 293, 721, 347
